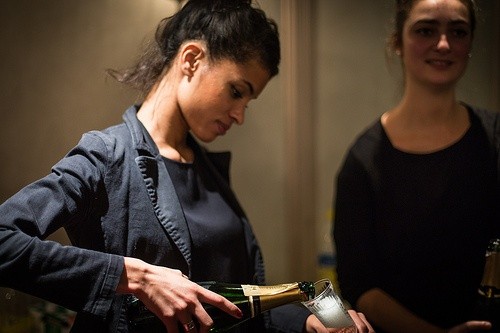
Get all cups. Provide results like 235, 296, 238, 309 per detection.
301, 279, 357, 333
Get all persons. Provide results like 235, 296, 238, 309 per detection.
0, 0, 375, 333
329, 0, 500, 333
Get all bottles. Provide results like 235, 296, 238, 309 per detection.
125, 279, 316, 333
474, 241, 499, 333
